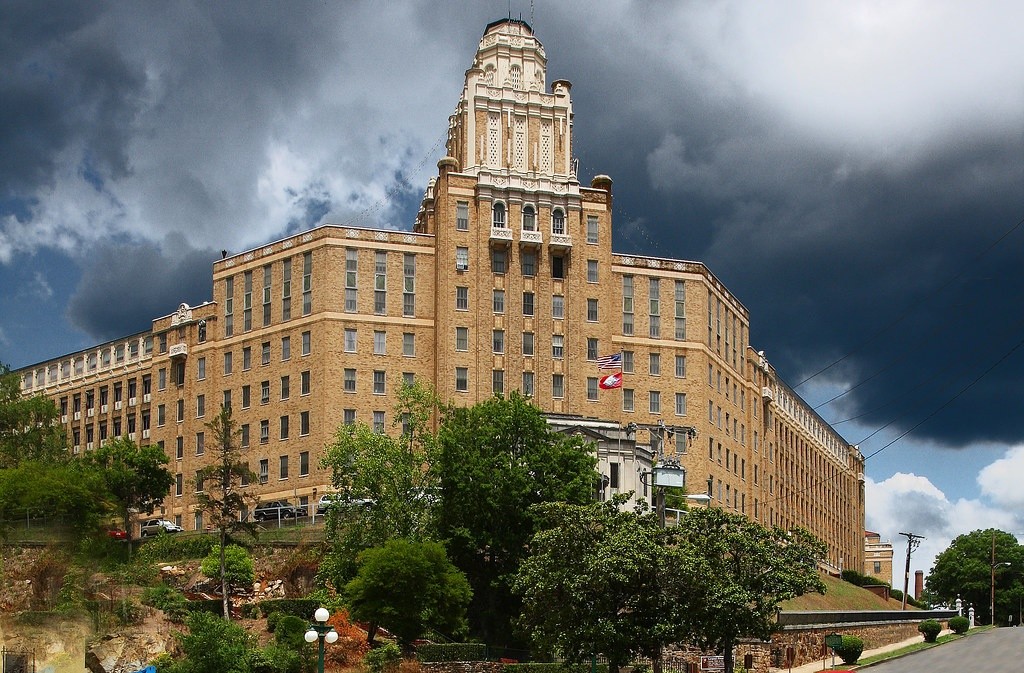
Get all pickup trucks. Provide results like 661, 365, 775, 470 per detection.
317, 494, 373, 513
252, 500, 308, 521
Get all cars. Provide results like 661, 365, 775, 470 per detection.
100, 524, 126, 540
407, 486, 444, 505
141, 518, 184, 537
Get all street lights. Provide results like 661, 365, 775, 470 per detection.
991, 561, 1012, 626
301, 608, 339, 673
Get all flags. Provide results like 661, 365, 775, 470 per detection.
599, 371, 622, 389
597, 352, 621, 370
